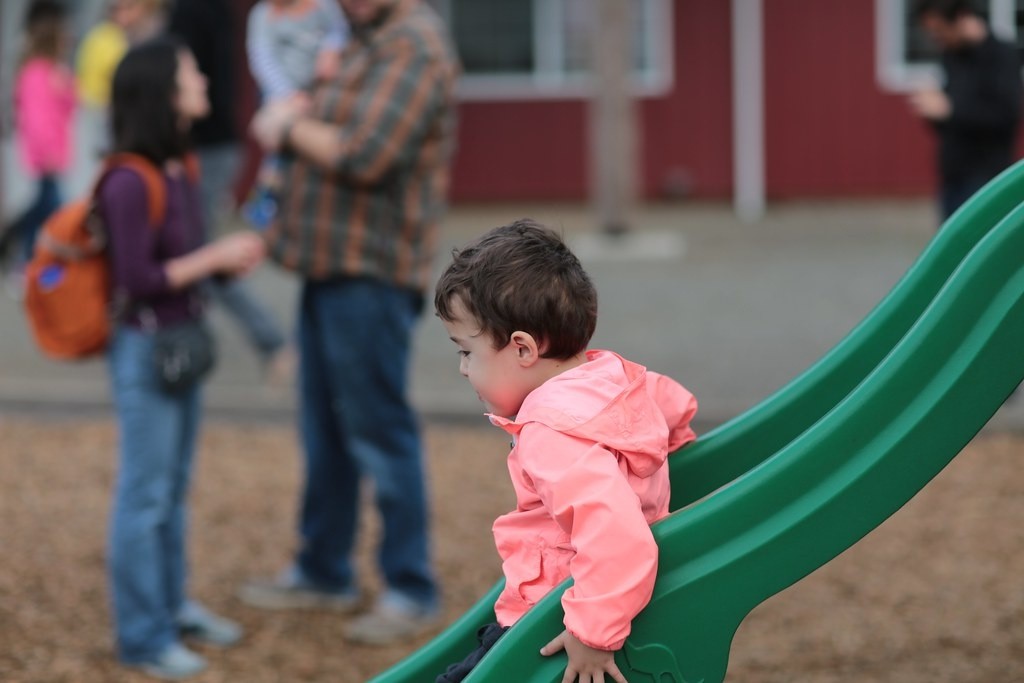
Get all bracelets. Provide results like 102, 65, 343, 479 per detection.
278, 120, 296, 159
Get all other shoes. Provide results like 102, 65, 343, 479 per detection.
135, 638, 207, 678
174, 607, 244, 647
342, 593, 441, 645
237, 567, 359, 612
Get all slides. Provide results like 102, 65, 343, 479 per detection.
356, 156, 1022, 681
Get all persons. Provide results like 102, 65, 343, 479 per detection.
427, 219, 699, 683
234, 0, 465, 646
91, 41, 264, 677
1, 1, 350, 387
910, 0, 1023, 224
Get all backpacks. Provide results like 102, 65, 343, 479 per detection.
24, 151, 200, 362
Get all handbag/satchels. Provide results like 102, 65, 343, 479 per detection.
150, 323, 214, 392
